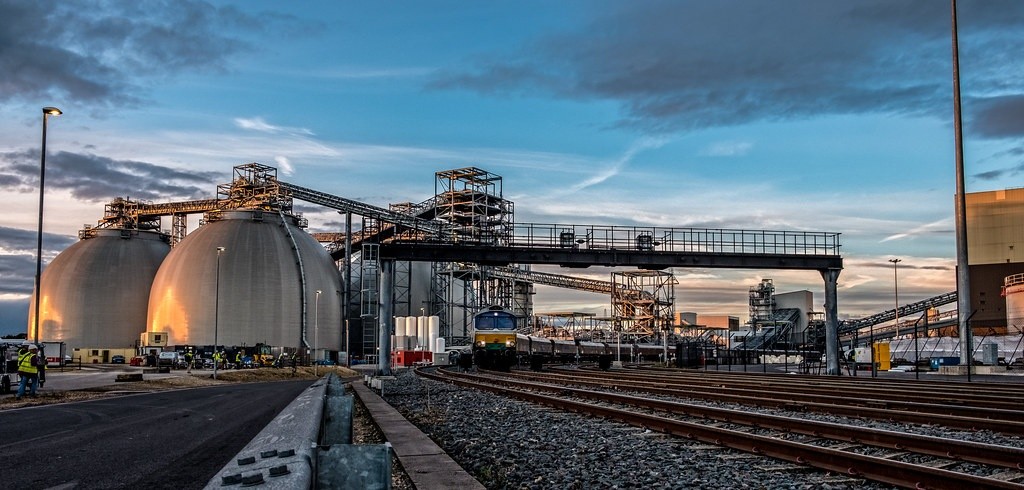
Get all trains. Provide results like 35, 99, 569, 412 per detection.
470, 304, 725, 371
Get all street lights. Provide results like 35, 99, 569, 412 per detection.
420, 307, 425, 366
888, 258, 902, 339
213, 247, 226, 379
33, 106, 63, 344
314, 289, 323, 376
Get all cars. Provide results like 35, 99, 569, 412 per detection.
159, 350, 254, 368
111, 354, 125, 364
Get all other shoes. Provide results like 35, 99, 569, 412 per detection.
30, 396, 39, 399
187, 371, 191, 373
16, 397, 22, 401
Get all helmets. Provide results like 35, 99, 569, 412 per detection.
21, 341, 30, 346
189, 350, 192, 353
28, 345, 38, 349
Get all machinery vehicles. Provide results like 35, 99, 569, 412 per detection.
253, 344, 274, 367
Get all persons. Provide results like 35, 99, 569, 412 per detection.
15, 344, 41, 400
37, 346, 48, 387
278, 354, 284, 368
17, 341, 31, 367
291, 352, 296, 370
185, 348, 243, 373
